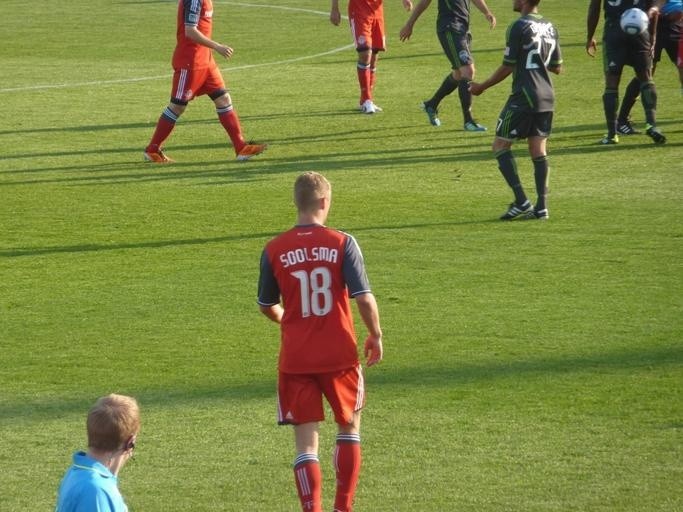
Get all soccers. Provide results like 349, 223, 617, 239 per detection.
620, 8, 649, 35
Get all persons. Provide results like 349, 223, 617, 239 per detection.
331, 0, 413, 113
143, 0, 266, 163
55, 394, 139, 512
587, 0, 667, 144
467, 0, 563, 220
616, 0, 683, 135
399, 0, 496, 131
258, 172, 383, 512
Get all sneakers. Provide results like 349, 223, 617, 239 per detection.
592, 133, 619, 144
464, 118, 487, 131
422, 101, 441, 126
143, 146, 175, 164
615, 115, 642, 135
359, 99, 381, 114
500, 199, 534, 220
520, 208, 549, 219
644, 121, 667, 145
235, 136, 267, 161
